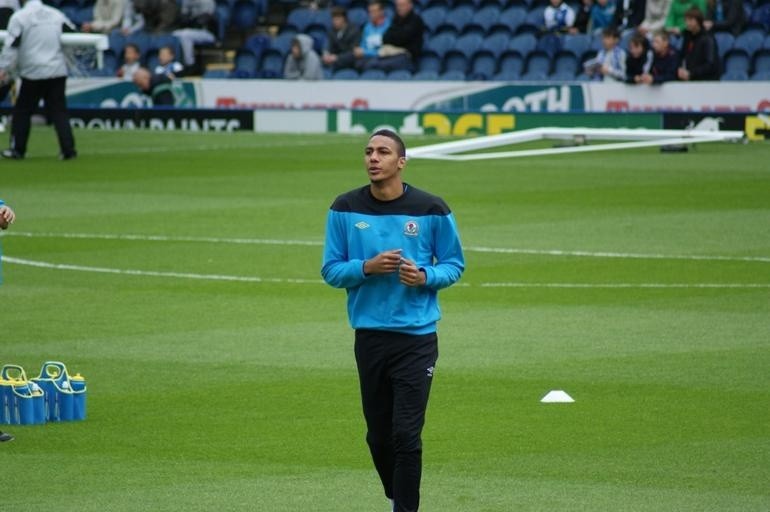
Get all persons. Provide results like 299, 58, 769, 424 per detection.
283, 34, 324, 79
319, 6, 362, 76
353, 0, 390, 74
363, 0, 424, 75
321, 129, 466, 510
545, 0, 747, 151
0, 200, 14, 445
0, 1, 218, 162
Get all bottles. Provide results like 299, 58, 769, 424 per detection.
13, 380, 32, 427
70, 371, 86, 421
57, 381, 73, 422
31, 382, 45, 426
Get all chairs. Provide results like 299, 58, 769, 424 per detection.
280, 2, 770, 23
201, 46, 770, 80
105, 31, 192, 50
230, 23, 768, 46
100, 49, 185, 76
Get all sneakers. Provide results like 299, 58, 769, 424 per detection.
1, 150, 24, 159
57, 151, 76, 161
